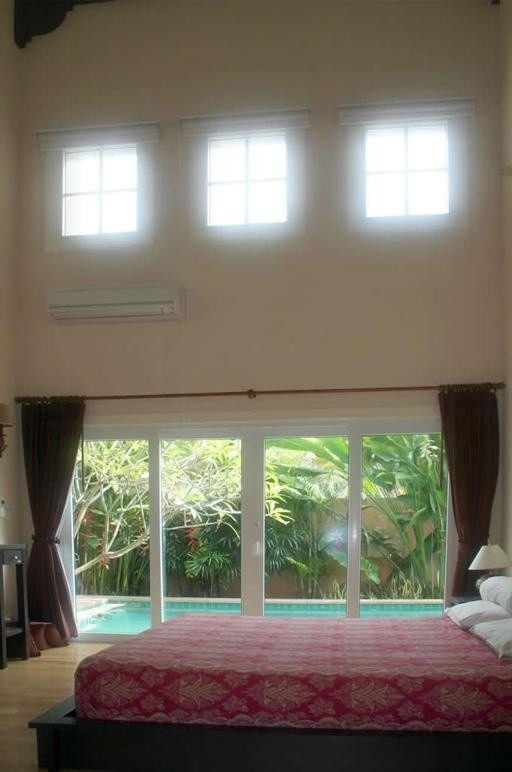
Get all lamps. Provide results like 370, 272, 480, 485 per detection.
468, 537, 512, 595
0, 402, 13, 458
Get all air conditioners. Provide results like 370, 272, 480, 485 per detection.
44, 282, 187, 326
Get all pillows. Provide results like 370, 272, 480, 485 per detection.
480, 576, 512, 614
469, 618, 512, 660
445, 600, 512, 630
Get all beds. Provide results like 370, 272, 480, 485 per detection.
27, 610, 512, 772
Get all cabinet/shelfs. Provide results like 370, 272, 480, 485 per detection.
0, 543, 31, 669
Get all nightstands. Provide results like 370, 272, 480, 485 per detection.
448, 595, 481, 610
